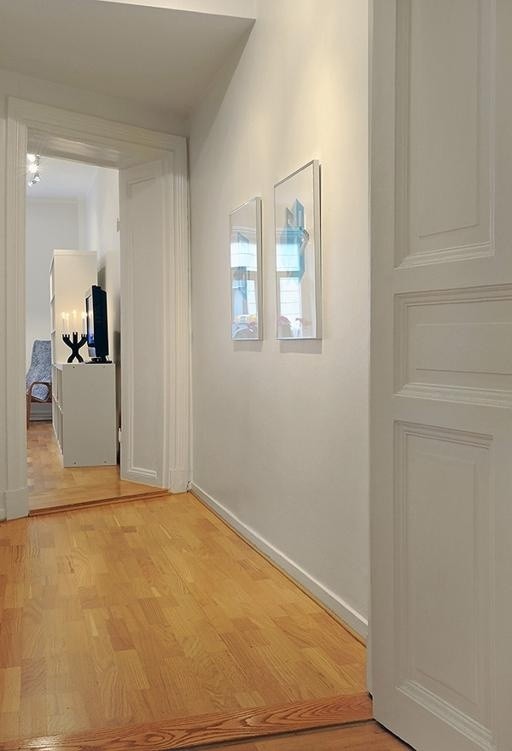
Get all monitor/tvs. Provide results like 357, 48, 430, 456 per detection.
84, 285, 112, 364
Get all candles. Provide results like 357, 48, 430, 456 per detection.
60, 307, 88, 335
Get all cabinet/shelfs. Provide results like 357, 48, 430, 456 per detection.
48, 249, 98, 434
55, 363, 117, 468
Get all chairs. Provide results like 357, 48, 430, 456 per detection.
26, 339, 51, 430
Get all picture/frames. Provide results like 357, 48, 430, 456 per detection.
228, 197, 263, 341
273, 160, 324, 342
